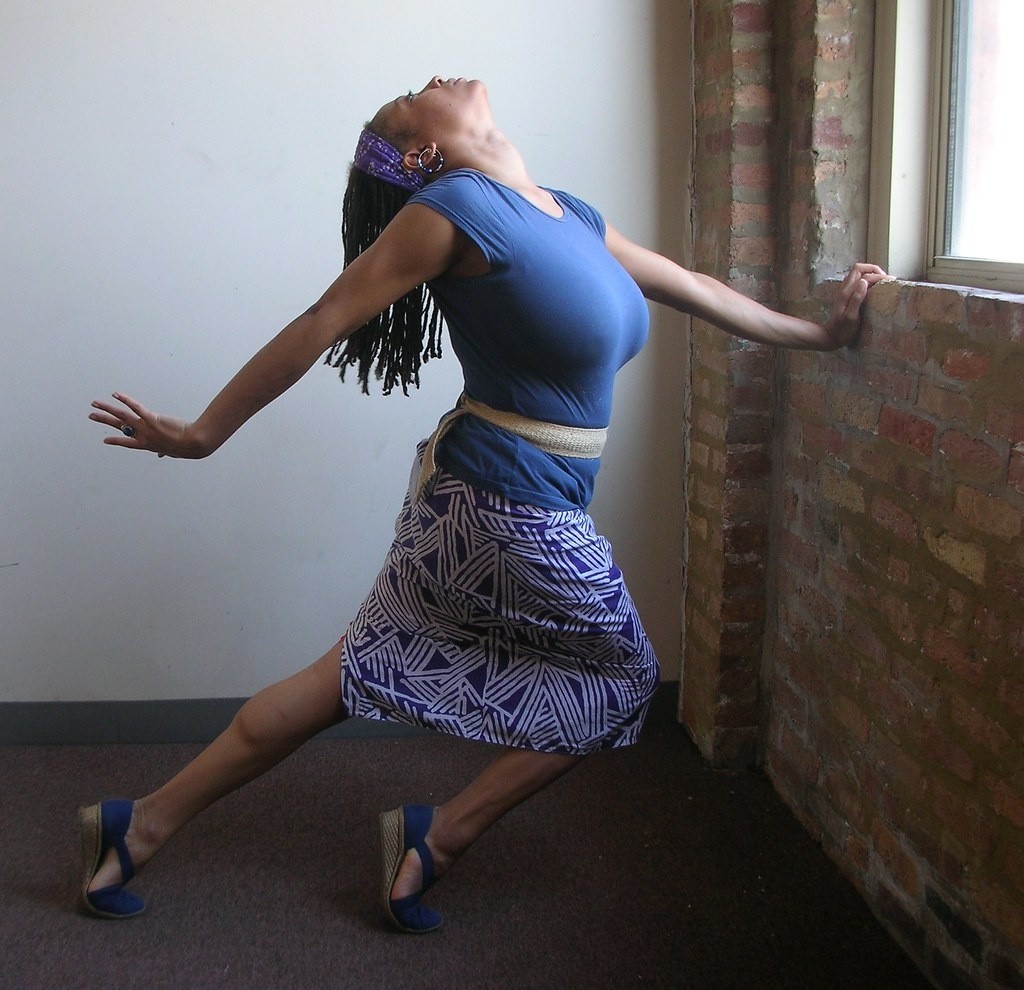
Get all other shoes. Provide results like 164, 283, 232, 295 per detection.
380, 803, 444, 932
81, 800, 144, 917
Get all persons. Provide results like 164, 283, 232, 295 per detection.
77, 76, 899, 933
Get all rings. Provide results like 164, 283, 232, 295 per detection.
121, 424, 137, 437
